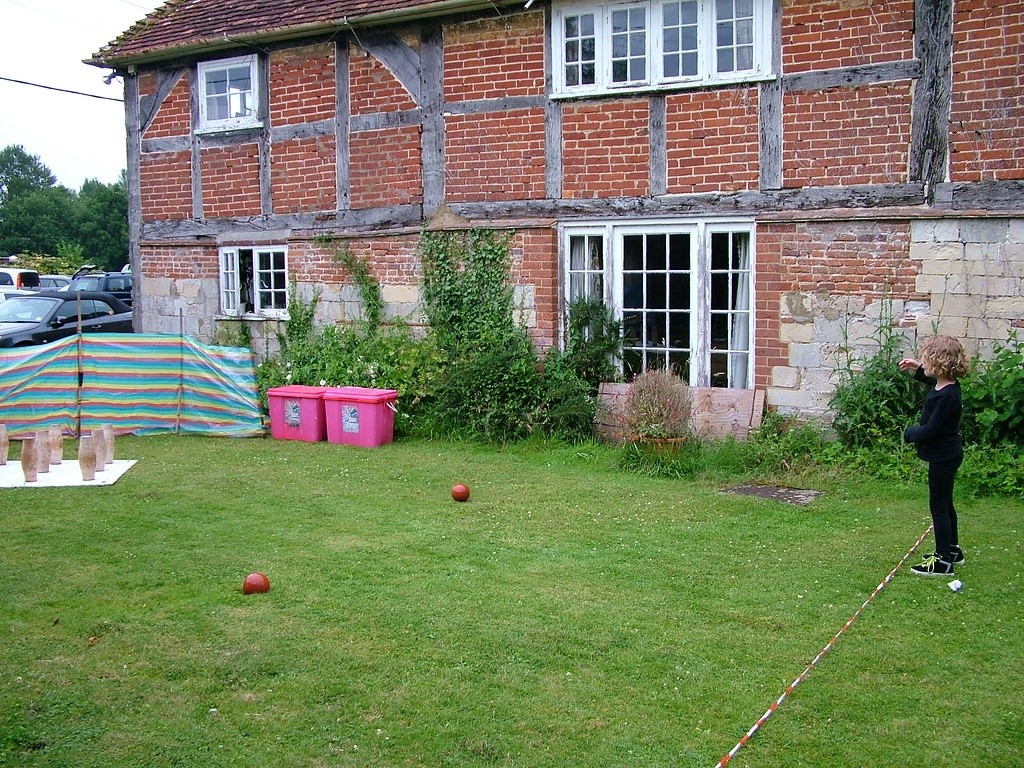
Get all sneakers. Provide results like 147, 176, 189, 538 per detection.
922, 544, 965, 565
910, 551, 954, 576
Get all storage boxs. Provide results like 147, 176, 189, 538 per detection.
324, 386, 400, 447
266, 385, 337, 442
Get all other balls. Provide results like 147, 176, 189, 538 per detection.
243, 573, 270, 595
451, 483, 470, 502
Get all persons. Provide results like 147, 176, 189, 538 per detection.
899, 336, 969, 574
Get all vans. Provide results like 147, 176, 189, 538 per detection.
0, 267, 42, 292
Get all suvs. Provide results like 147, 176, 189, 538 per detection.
68, 272, 134, 308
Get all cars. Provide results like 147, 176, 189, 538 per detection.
0, 289, 134, 349
0, 263, 131, 304
